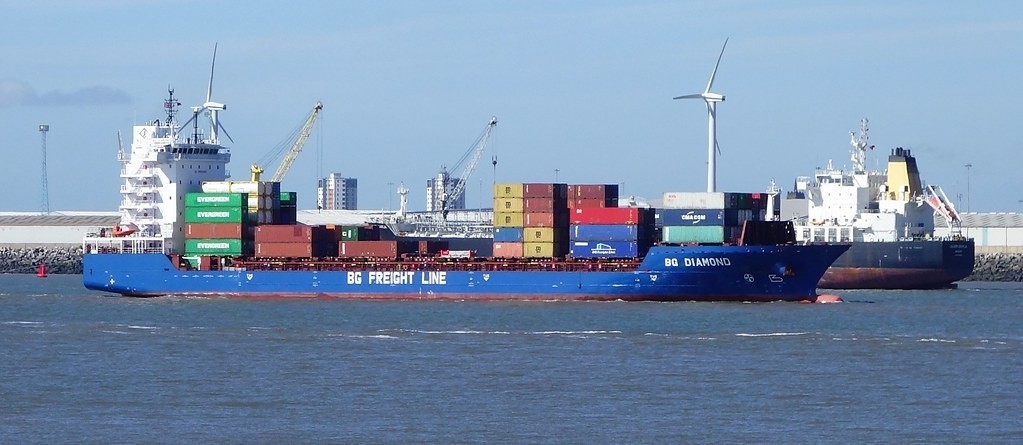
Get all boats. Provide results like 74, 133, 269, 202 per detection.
78, 163, 856, 305
765, 116, 980, 287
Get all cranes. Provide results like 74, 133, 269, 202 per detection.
253, 99, 324, 183
416, 115, 501, 227
